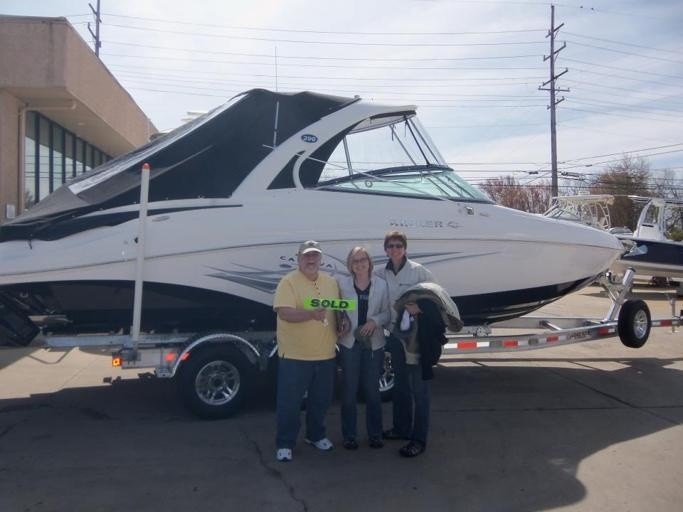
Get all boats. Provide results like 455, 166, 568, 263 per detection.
0, 87, 625, 349
608, 193, 683, 281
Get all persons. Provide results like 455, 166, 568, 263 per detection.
335, 246, 391, 450
382, 230, 441, 458
273, 241, 350, 464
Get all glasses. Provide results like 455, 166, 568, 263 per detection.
353, 257, 367, 264
387, 244, 403, 248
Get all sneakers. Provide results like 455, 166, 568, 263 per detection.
305, 438, 333, 451
368, 438, 383, 448
277, 448, 292, 461
384, 429, 409, 439
400, 440, 424, 456
344, 438, 357, 449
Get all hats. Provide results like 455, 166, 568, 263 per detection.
299, 241, 321, 255
